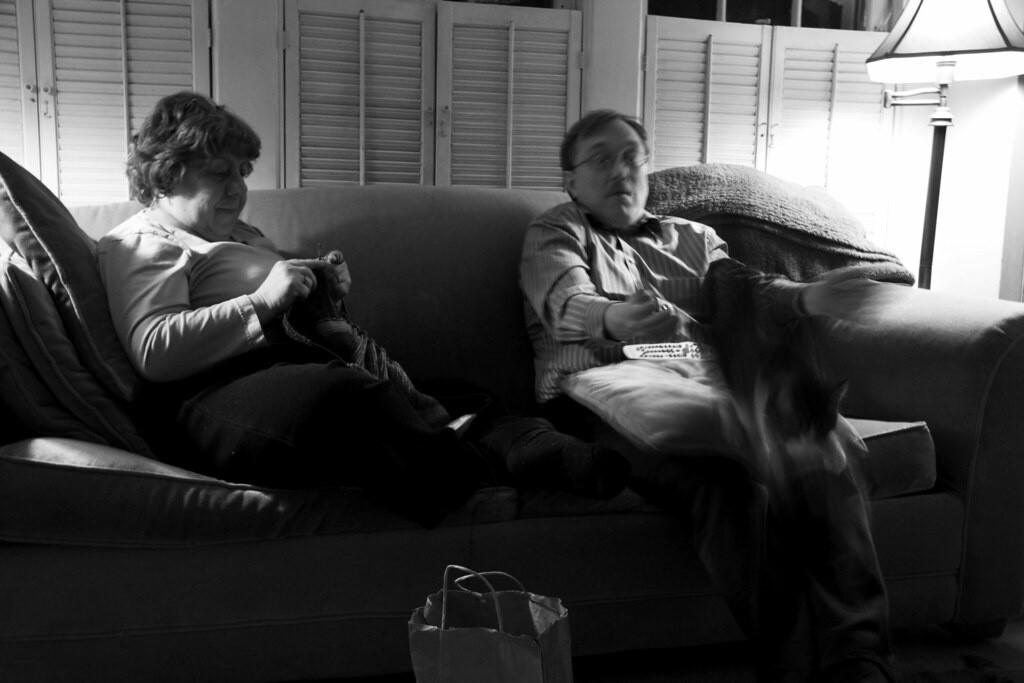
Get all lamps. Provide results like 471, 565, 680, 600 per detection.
866, 0, 1024, 291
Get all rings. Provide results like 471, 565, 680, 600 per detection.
304, 275, 308, 284
335, 275, 340, 283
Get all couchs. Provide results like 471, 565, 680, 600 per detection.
0, 186, 1024, 683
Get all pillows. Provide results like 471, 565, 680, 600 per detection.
561, 361, 748, 459
0, 151, 168, 461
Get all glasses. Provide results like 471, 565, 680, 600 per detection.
572, 151, 647, 170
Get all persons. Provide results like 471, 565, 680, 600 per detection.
521, 109, 895, 683
97, 92, 630, 530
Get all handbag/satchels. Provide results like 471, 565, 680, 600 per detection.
409, 564, 573, 683
